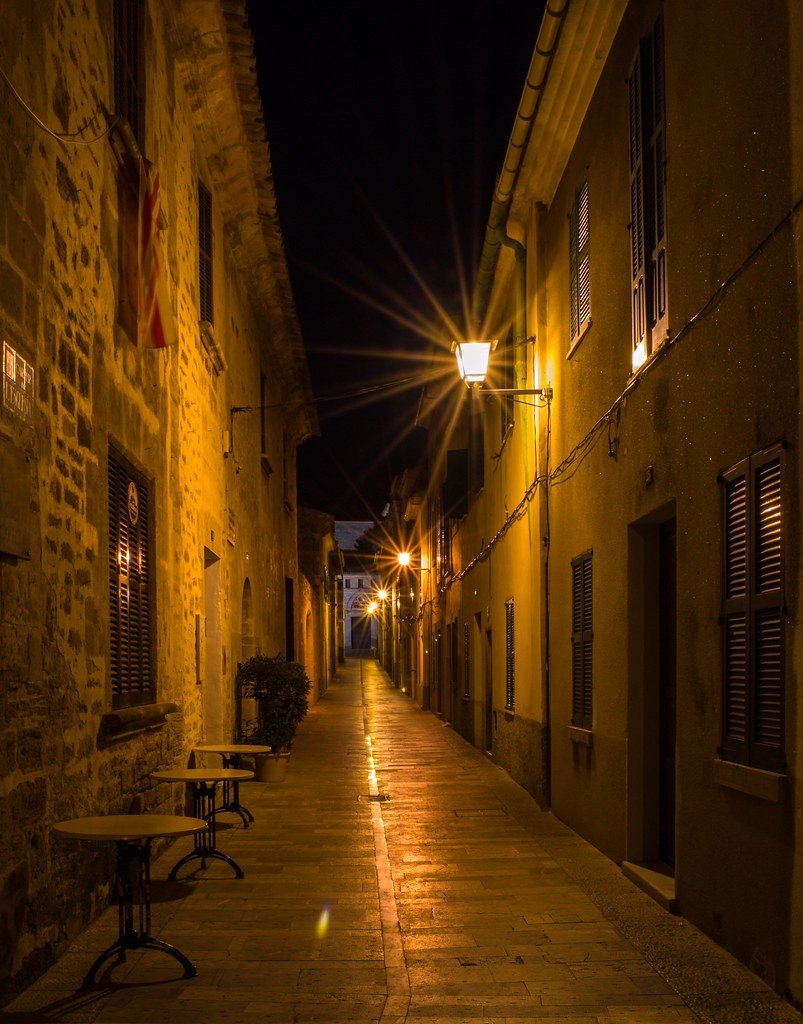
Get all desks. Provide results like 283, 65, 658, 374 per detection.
193, 743, 272, 828
52, 814, 211, 995
151, 768, 256, 884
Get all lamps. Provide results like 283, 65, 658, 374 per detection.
397, 550, 429, 573
448, 319, 553, 400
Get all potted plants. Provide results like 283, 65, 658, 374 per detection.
237, 653, 313, 784
